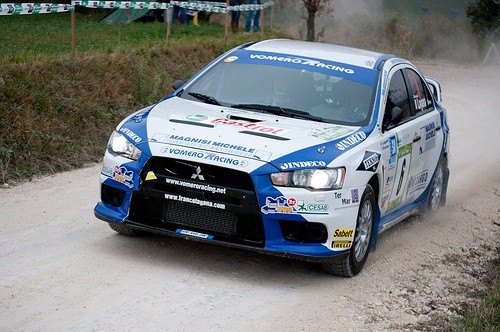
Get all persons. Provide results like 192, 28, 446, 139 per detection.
228, 0, 244, 35
268, 77, 296, 108
173, 3, 189, 25
331, 82, 368, 122
244, 0, 263, 32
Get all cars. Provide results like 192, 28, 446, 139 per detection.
94, 39, 450, 278
